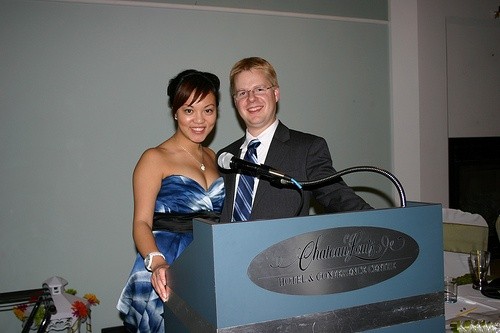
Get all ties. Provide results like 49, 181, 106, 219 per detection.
233, 139, 261, 222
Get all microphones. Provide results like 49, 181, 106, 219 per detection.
218, 152, 406, 218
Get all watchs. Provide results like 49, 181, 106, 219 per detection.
144, 252, 165, 271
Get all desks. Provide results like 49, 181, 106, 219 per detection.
444, 282, 500, 333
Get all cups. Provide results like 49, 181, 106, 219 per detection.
444, 276, 458, 304
470, 250, 490, 291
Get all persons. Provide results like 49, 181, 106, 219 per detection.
215, 57, 374, 223
116, 69, 225, 333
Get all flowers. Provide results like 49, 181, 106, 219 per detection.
11, 289, 100, 328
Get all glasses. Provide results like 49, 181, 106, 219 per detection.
233, 85, 275, 101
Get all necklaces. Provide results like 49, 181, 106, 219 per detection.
172, 136, 206, 171
